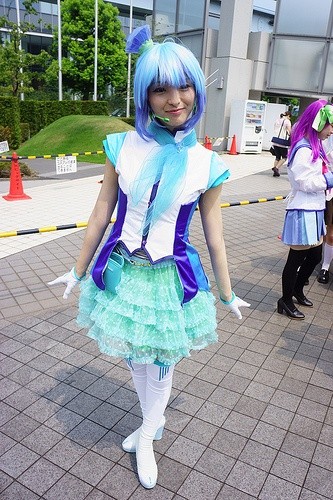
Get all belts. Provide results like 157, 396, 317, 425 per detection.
119, 242, 148, 259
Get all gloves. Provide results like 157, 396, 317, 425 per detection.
48, 265, 87, 299
219, 290, 250, 320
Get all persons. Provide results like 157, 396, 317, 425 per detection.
48, 42, 252, 489
277, 100, 333, 321
270, 110, 292, 177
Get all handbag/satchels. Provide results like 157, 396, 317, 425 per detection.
271, 136, 290, 146
270, 147, 277, 156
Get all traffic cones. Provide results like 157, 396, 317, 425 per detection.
227, 135, 239, 155
2, 151, 31, 202
205, 135, 213, 151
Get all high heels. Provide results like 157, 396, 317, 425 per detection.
136, 436, 157, 489
278, 298, 305, 320
122, 416, 166, 453
293, 291, 313, 307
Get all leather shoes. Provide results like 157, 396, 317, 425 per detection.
317, 269, 329, 284
297, 272, 309, 285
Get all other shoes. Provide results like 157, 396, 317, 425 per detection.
273, 173, 280, 177
272, 167, 279, 175
